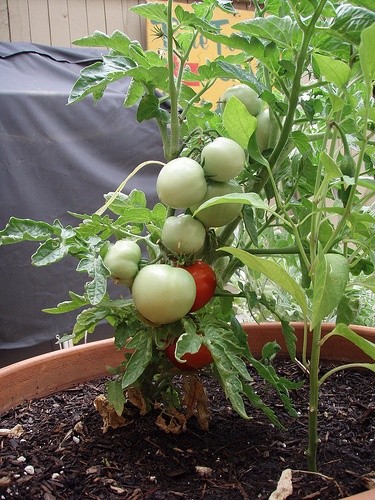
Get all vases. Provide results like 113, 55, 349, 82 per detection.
0, 325, 375, 499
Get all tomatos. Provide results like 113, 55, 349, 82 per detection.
104, 82, 283, 325
163, 337, 213, 371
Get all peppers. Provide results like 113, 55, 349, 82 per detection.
332, 122, 356, 208
